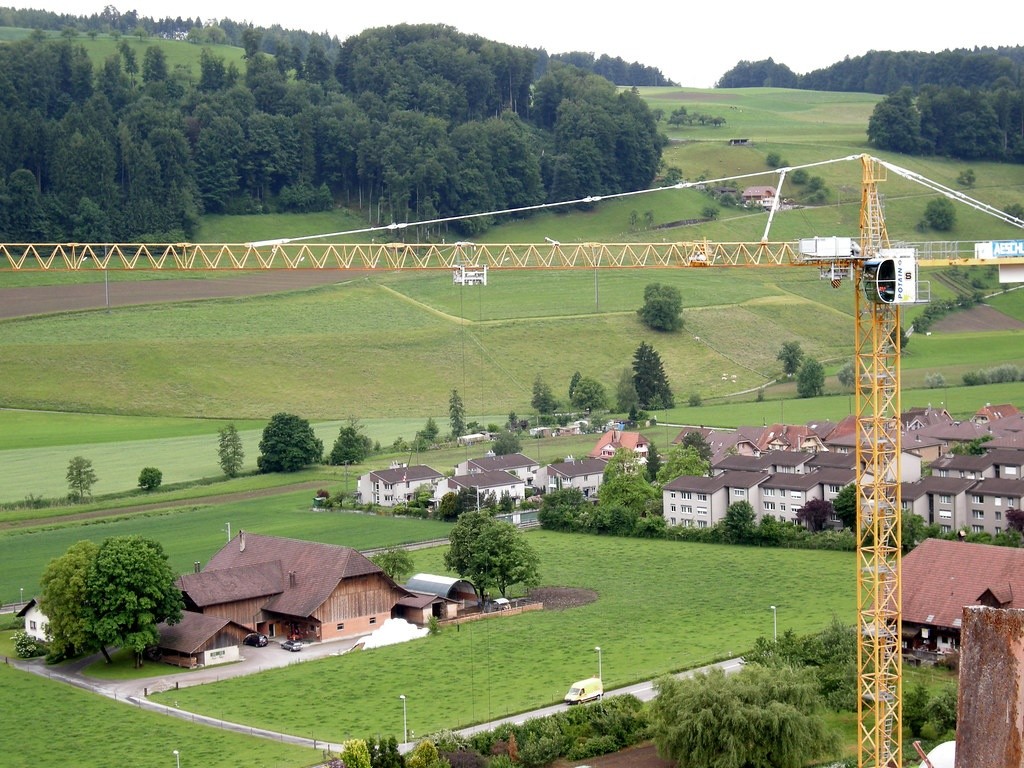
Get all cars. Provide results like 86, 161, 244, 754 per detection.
287, 631, 304, 641
244, 633, 268, 647
281, 640, 304, 653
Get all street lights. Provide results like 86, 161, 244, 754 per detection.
19, 588, 24, 607
400, 695, 408, 754
173, 750, 181, 768
770, 605, 777, 645
595, 646, 604, 702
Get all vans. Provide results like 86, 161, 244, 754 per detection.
564, 677, 605, 707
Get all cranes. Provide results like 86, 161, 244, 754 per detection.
0, 155, 1023, 766
